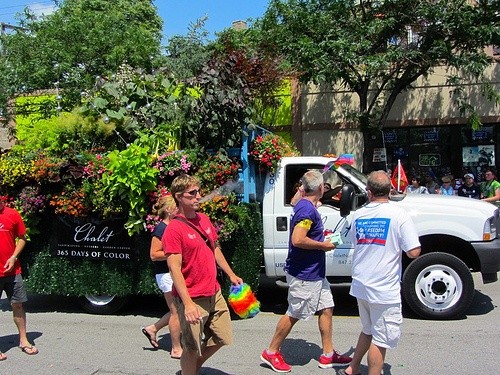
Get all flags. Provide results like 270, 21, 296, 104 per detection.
391, 165, 408, 193
321, 154, 353, 170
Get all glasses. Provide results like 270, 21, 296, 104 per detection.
442, 182, 449, 184
184, 188, 201, 195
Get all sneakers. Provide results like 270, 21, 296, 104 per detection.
318, 348, 353, 368
260, 348, 292, 372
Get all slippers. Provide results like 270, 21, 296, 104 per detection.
142, 329, 159, 350
337, 368, 349, 375
19, 345, 39, 355
0, 350, 7, 361
171, 355, 181, 360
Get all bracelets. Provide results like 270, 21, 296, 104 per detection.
12, 255, 18, 260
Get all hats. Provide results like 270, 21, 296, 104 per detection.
464, 173, 474, 180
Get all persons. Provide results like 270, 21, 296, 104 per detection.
481, 170, 500, 205
142, 195, 184, 359
260, 170, 352, 373
0, 191, 39, 361
407, 176, 429, 194
340, 171, 421, 375
434, 175, 455, 195
458, 174, 481, 200
162, 174, 243, 375
316, 183, 340, 206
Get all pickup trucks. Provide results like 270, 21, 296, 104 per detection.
23, 155, 500, 320
